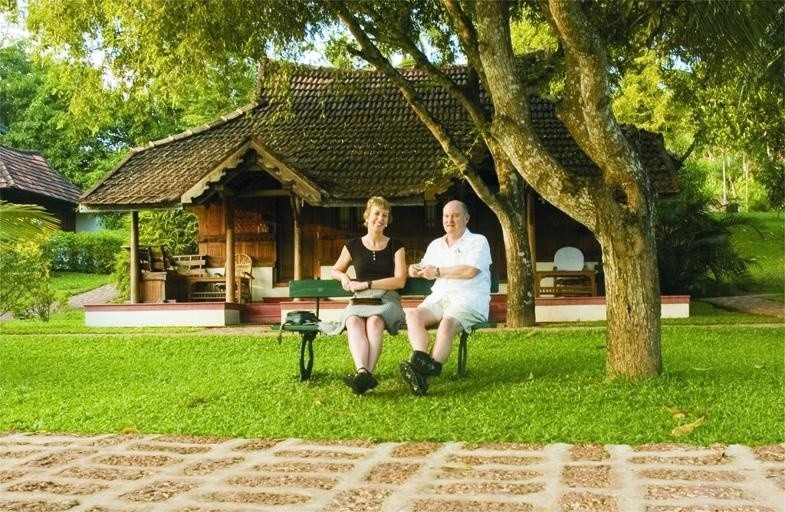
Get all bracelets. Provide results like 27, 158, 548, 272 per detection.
368, 280, 372, 289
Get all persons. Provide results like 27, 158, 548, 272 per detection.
399, 199, 494, 398
330, 197, 407, 395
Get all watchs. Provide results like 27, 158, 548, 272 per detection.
435, 267, 440, 278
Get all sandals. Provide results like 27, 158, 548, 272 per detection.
343, 367, 379, 395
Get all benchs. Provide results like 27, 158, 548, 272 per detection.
280, 277, 497, 381
120, 244, 208, 303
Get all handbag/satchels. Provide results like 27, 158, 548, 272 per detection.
286, 311, 319, 325
350, 298, 383, 305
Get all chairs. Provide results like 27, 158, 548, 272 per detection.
222, 255, 252, 279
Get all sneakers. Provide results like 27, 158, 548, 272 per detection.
410, 350, 443, 377
399, 360, 430, 396
